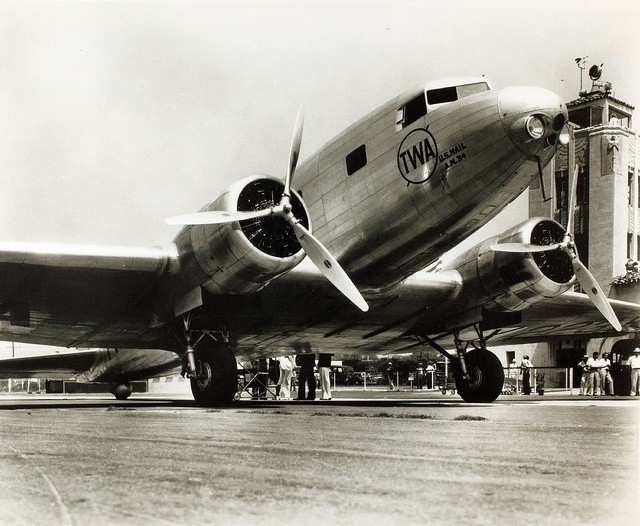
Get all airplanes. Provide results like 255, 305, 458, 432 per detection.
0, 73, 639, 410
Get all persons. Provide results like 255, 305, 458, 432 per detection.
578, 355, 592, 397
600, 353, 615, 396
522, 357, 533, 397
386, 363, 395, 390
586, 352, 601, 396
252, 360, 268, 401
295, 357, 318, 401
425, 361, 436, 391
316, 353, 332, 400
509, 360, 518, 380
521, 356, 529, 373
415, 363, 425, 390
626, 348, 640, 396
